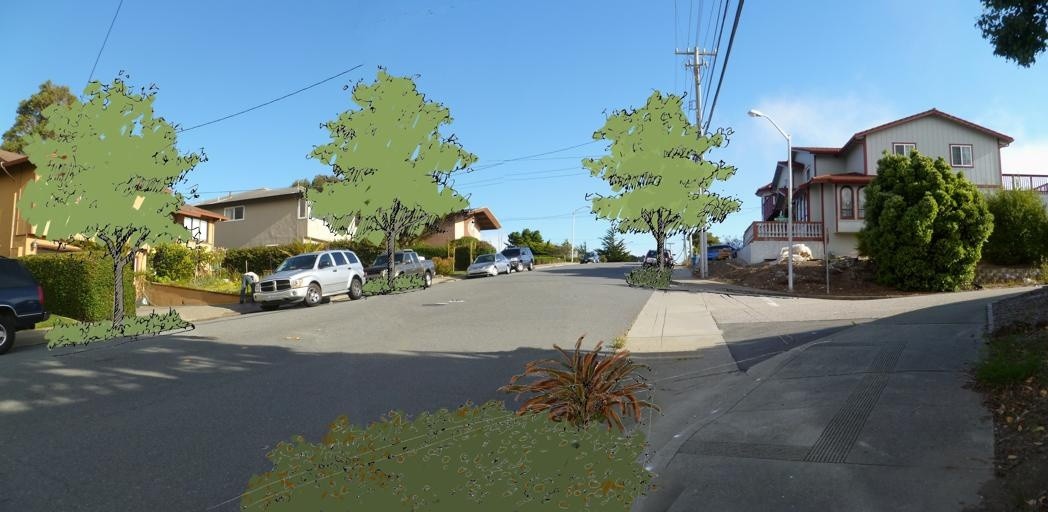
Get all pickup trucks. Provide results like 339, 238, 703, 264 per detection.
365, 249, 436, 288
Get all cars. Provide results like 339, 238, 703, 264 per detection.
708, 244, 737, 262
467, 253, 511, 277
397, 249, 426, 263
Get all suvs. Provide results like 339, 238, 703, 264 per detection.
501, 246, 535, 273
0, 254, 49, 354
252, 248, 367, 306
644, 250, 675, 271
579, 251, 600, 264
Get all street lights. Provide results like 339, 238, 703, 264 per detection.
570, 205, 592, 262
747, 109, 796, 291
